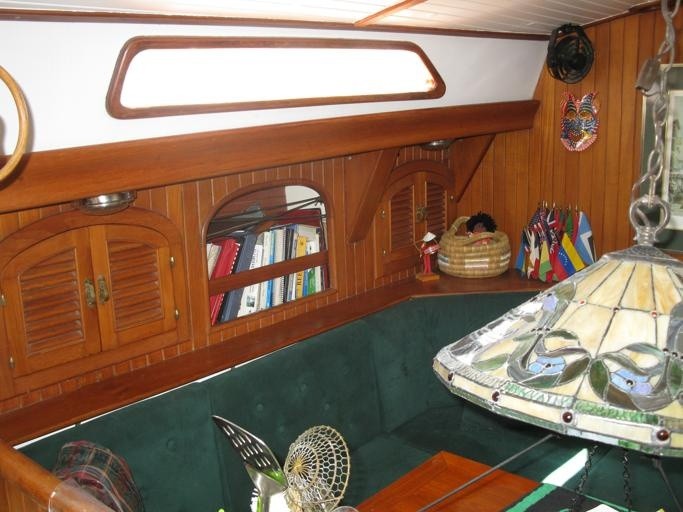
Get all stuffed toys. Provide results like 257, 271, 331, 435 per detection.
454, 210, 498, 247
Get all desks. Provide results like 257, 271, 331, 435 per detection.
349, 450, 633, 512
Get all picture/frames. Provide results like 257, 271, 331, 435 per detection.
634, 62, 682, 255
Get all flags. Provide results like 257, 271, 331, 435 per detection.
515, 201, 597, 282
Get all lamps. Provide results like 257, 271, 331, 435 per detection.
430, 0, 683, 461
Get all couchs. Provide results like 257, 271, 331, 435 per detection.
15, 291, 683, 512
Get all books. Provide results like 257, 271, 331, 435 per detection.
205, 208, 330, 327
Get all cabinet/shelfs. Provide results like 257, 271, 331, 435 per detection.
0, 100, 540, 446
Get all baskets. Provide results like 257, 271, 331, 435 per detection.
436, 216, 511, 279
